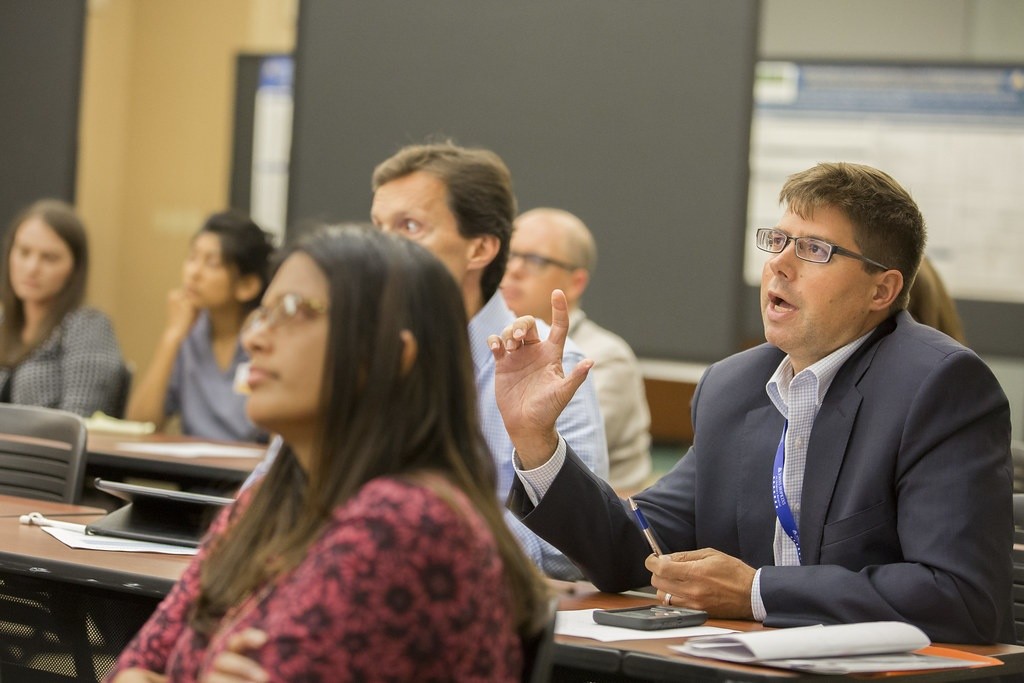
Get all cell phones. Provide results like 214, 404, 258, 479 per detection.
593, 604, 708, 630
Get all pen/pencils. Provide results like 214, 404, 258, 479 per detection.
627, 495, 662, 557
20, 515, 89, 535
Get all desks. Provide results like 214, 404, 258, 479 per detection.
0, 418, 1024, 683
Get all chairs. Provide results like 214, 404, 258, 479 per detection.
0, 400, 103, 683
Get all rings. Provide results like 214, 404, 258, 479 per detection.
665, 594, 672, 606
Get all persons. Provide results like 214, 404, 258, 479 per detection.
499, 207, 653, 492
236, 143, 608, 583
909, 255, 964, 344
128, 212, 277, 446
486, 164, 1016, 644
0, 198, 123, 419
102, 222, 546, 683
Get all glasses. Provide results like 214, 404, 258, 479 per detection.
240, 294, 333, 333
755, 228, 889, 272
508, 251, 576, 275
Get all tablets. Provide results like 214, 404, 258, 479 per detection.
93, 478, 240, 507
